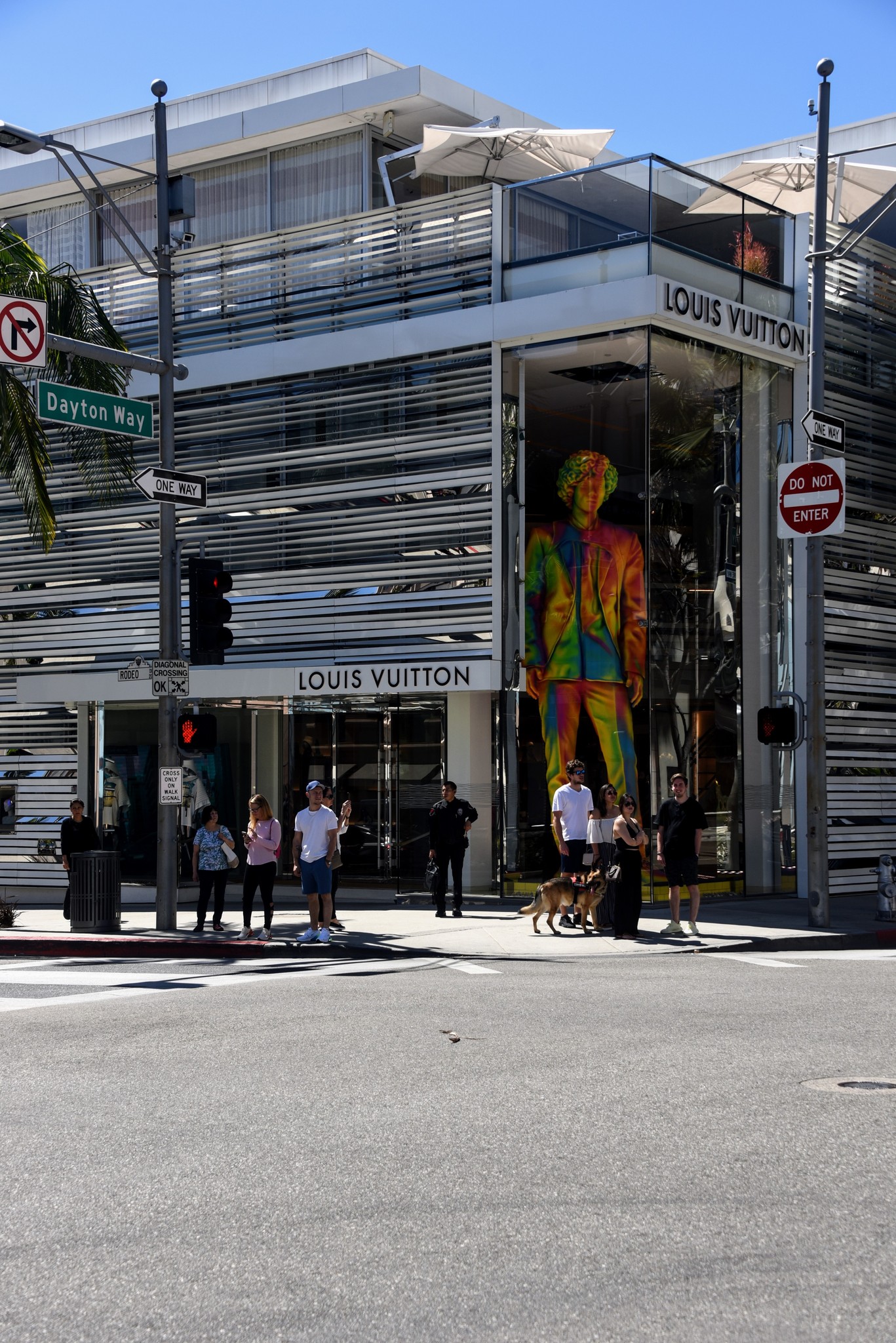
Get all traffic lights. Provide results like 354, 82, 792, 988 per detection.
186, 557, 234, 666
757, 705, 797, 745
178, 713, 218, 755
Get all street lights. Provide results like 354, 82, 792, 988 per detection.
0, 73, 186, 933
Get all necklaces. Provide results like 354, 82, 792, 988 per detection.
308, 809, 319, 816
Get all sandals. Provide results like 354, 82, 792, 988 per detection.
559, 912, 643, 939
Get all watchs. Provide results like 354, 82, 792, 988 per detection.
325, 859, 331, 865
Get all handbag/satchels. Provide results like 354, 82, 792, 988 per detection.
605, 866, 622, 882
583, 852, 595, 865
219, 825, 239, 868
425, 858, 440, 891
331, 849, 343, 870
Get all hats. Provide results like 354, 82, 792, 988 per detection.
182, 760, 197, 776
306, 781, 325, 792
104, 761, 119, 776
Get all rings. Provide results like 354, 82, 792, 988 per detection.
347, 804, 349, 806
640, 839, 641, 840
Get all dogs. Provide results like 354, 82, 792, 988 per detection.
516, 861, 608, 934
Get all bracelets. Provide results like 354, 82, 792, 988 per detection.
657, 852, 662, 854
345, 815, 349, 819
696, 854, 698, 857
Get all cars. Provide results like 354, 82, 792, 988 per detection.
340, 824, 406, 873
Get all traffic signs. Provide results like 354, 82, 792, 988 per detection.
801, 408, 846, 453
132, 467, 207, 508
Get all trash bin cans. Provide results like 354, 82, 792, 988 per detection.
69, 850, 122, 933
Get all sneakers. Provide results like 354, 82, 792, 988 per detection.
237, 925, 254, 940
257, 928, 272, 940
316, 928, 332, 942
660, 919, 683, 933
296, 928, 318, 941
317, 927, 334, 934
329, 922, 346, 930
683, 921, 699, 935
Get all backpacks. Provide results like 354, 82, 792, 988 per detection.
270, 819, 280, 859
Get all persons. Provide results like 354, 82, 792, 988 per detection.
103, 761, 131, 826
192, 805, 235, 932
178, 759, 211, 837
586, 784, 621, 930
427, 781, 478, 917
61, 799, 101, 920
653, 773, 709, 935
612, 793, 649, 939
318, 786, 352, 932
292, 781, 338, 942
552, 760, 594, 928
521, 449, 647, 854
2, 799, 14, 824
237, 794, 281, 940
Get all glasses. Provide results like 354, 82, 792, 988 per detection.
323, 795, 333, 800
571, 770, 585, 775
606, 791, 618, 796
623, 801, 636, 807
249, 806, 261, 812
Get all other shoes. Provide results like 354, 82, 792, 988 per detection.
213, 924, 224, 931
436, 909, 446, 917
453, 907, 462, 917
63, 904, 71, 919
193, 924, 204, 931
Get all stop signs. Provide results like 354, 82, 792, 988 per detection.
779, 461, 843, 535
